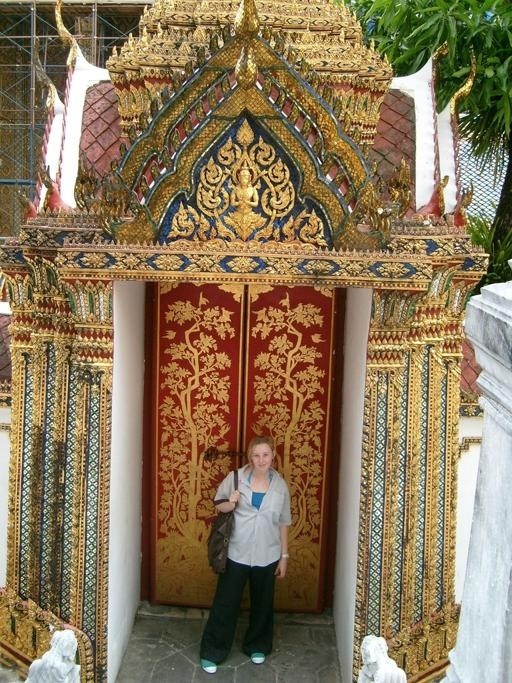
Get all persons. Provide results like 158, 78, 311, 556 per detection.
229, 164, 261, 218
198, 434, 292, 675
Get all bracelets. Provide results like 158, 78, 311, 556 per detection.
281, 553, 290, 559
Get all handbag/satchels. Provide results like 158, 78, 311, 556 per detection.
207, 469, 239, 575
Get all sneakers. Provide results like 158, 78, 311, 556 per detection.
250, 652, 266, 665
200, 657, 217, 673
